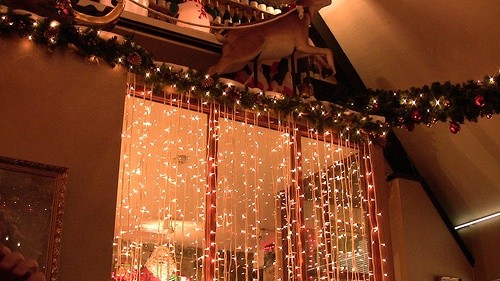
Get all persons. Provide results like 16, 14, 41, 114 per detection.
0, 243, 47, 281
116, 245, 191, 281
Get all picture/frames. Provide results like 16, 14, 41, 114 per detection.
0, 154, 70, 281
